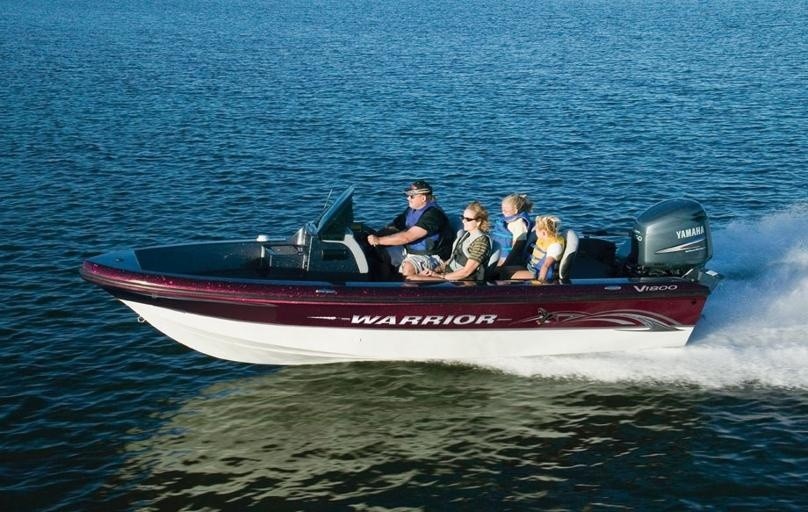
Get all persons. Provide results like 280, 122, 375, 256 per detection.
366, 179, 453, 279
509, 214, 567, 282
402, 200, 495, 283
487, 191, 533, 270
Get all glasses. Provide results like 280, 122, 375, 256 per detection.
461, 216, 477, 222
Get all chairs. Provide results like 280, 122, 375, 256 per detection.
558, 226, 581, 280
442, 227, 501, 287
499, 223, 540, 274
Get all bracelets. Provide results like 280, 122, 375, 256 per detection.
440, 271, 448, 278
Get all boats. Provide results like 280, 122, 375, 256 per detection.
75, 181, 715, 365
